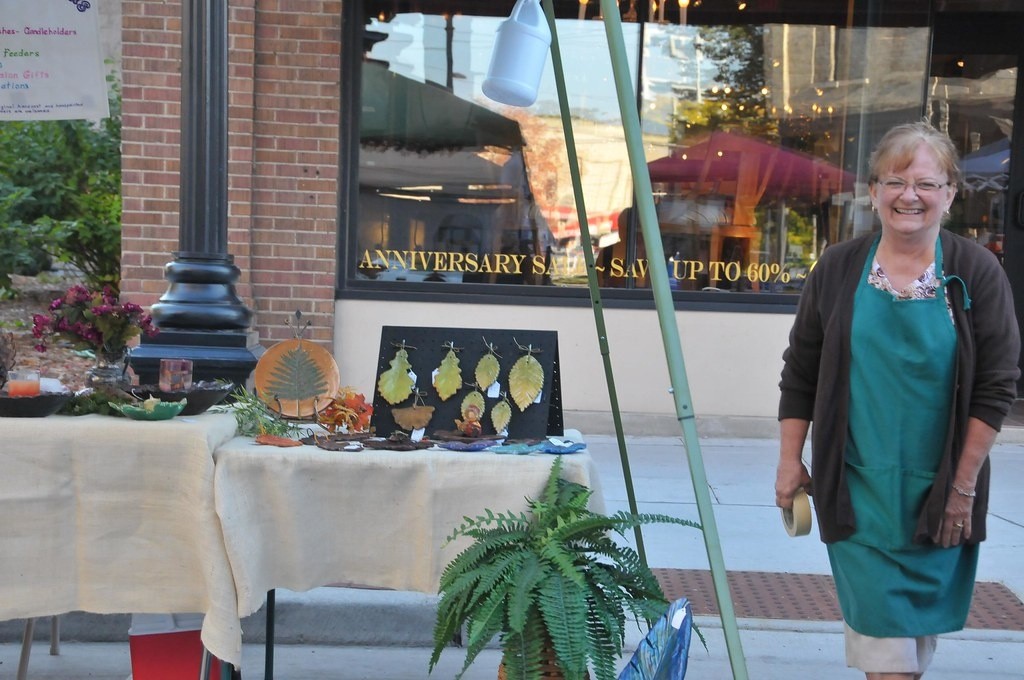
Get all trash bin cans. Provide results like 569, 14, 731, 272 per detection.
128, 611, 224, 680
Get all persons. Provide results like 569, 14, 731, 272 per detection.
775, 124, 1020, 680
984, 235, 1001, 252
595, 207, 646, 288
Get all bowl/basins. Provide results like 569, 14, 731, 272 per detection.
128, 381, 235, 416
0, 392, 74, 418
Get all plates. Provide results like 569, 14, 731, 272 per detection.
254, 431, 586, 454
255, 340, 340, 417
109, 400, 187, 420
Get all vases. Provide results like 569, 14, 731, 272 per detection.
84, 352, 132, 388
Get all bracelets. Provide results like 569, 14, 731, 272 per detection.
952, 483, 976, 497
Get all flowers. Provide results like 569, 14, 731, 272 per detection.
31, 284, 160, 364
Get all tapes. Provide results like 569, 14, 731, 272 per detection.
780, 490, 813, 538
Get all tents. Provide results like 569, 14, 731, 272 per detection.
352, 60, 555, 285
952, 139, 1011, 254
624, 130, 871, 290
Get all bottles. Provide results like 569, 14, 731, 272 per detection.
481, 0, 552, 106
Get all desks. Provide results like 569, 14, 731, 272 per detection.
0, 398, 262, 680
213, 420, 613, 680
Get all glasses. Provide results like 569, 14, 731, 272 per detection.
878, 176, 952, 192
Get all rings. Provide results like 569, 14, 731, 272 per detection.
954, 522, 964, 528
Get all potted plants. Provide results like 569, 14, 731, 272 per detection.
428, 454, 709, 679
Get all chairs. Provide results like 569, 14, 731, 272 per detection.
422, 135, 545, 284
624, 130, 778, 292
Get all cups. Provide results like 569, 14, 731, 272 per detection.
7, 371, 40, 398
159, 359, 193, 394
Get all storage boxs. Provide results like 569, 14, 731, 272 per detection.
127, 613, 221, 680
